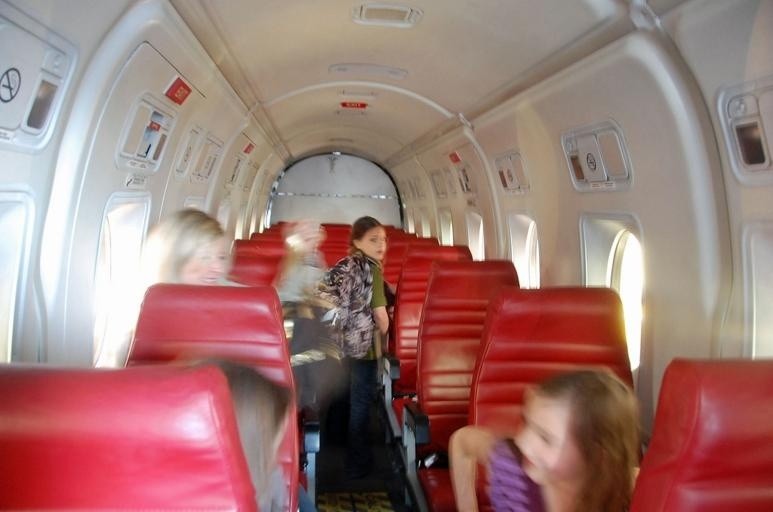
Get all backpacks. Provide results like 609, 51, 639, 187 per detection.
320, 253, 375, 363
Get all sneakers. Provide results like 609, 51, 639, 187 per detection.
342, 449, 401, 480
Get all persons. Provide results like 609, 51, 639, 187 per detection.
447, 365, 641, 512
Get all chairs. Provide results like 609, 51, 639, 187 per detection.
382, 245, 773, 512
1, 284, 321, 512
226, 222, 439, 285
